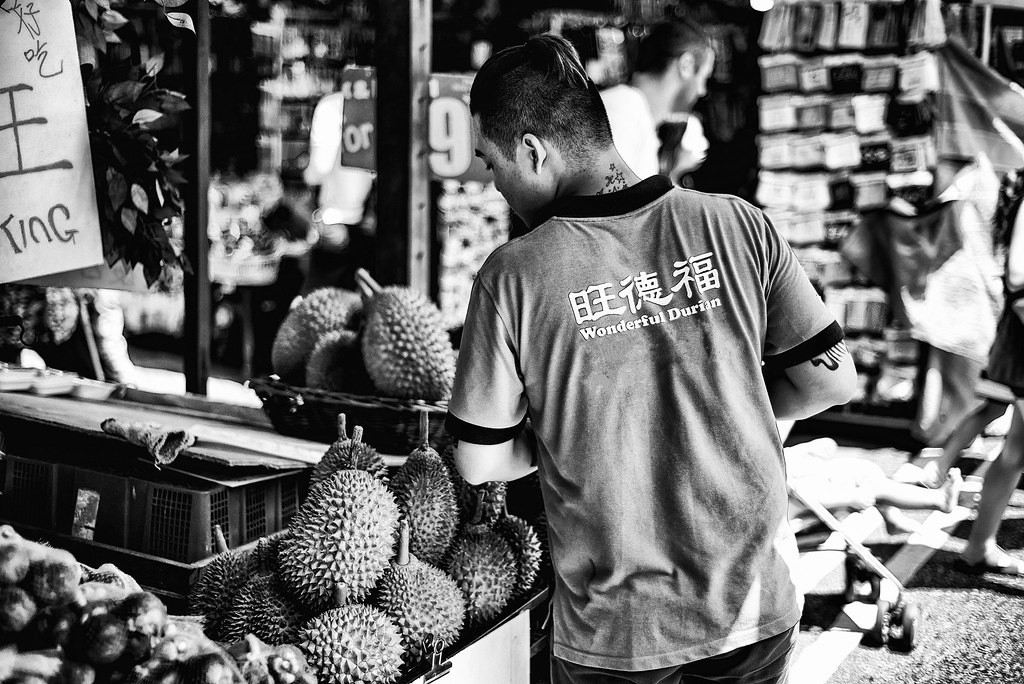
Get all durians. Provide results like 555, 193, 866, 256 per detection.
186, 412, 544, 684
272, 267, 457, 405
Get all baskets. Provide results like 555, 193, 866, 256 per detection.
1, 449, 302, 566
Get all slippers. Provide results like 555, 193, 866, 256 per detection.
956, 548, 1024, 575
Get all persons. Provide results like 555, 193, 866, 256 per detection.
653, 107, 710, 185
444, 30, 863, 684
598, 13, 723, 194
919, 153, 1011, 489
951, 166, 1024, 575
779, 437, 965, 515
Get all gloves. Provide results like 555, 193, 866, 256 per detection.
102, 419, 198, 467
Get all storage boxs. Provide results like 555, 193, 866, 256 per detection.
0, 522, 294, 616
0, 430, 312, 564
